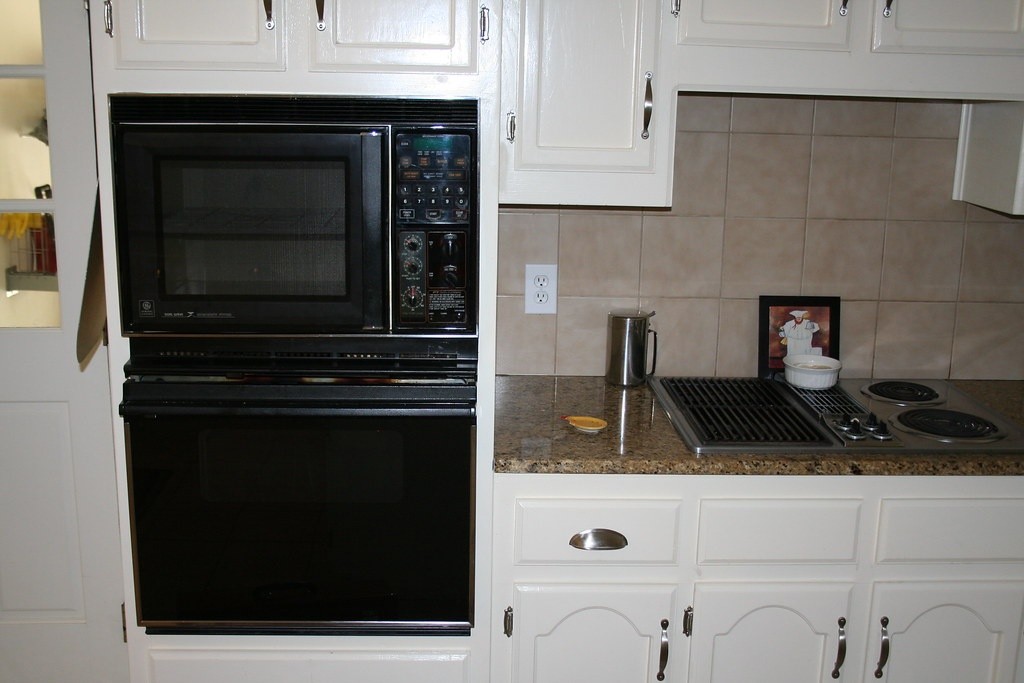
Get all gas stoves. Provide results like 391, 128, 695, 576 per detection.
649, 374, 1024, 459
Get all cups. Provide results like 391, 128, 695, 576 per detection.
605, 310, 659, 389
561, 416, 607, 432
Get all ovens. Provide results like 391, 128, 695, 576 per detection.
115, 381, 477, 639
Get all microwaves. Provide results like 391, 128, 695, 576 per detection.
107, 91, 481, 376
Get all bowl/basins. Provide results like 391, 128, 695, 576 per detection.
782, 354, 842, 389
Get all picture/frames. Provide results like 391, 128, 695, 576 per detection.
758, 295, 841, 377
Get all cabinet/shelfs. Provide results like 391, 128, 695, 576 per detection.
679, 0, 1024, 101
497, 0, 674, 209
491, 475, 1024, 683
87, 0, 501, 98
0, 0, 135, 682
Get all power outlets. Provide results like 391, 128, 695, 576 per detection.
524, 263, 557, 314
